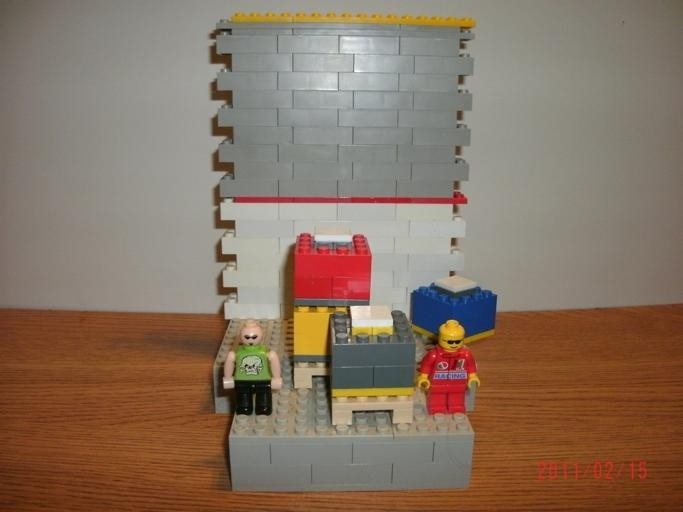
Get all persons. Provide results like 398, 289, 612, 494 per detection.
223, 320, 282, 415
415, 319, 480, 414
